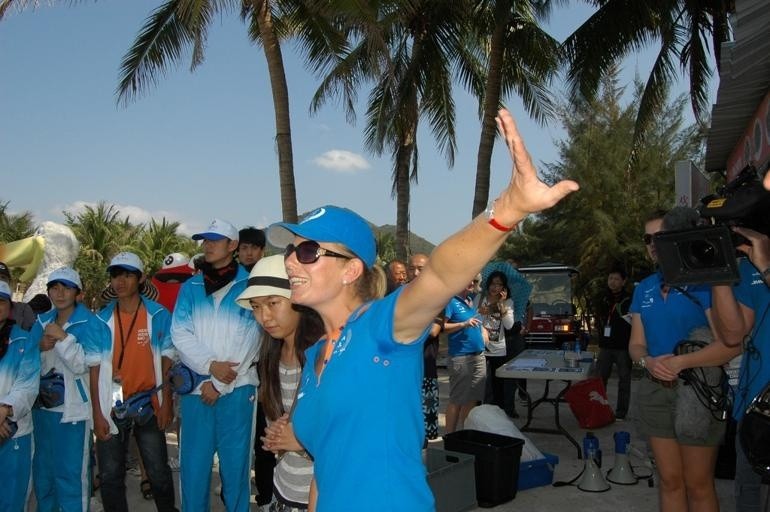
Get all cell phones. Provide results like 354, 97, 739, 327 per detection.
500, 287, 506, 295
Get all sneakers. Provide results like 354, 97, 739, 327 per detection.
125, 464, 142, 476
167, 457, 181, 471
89, 498, 104, 511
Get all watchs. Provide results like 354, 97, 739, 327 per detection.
486, 197, 515, 232
638, 355, 652, 368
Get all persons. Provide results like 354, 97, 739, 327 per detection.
707, 168, 769, 511
594, 268, 633, 422
627, 210, 742, 510
264, 110, 582, 512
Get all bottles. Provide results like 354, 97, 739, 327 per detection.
562, 342, 571, 354
115, 399, 126, 420
574, 337, 582, 353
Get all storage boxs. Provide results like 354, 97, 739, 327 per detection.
443, 431, 521, 507
516, 456, 558, 485
423, 448, 478, 510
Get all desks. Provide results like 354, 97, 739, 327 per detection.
496, 348, 598, 460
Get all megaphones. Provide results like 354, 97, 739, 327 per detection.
606, 431, 639, 485
577, 432, 611, 493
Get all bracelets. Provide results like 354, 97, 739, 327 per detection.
759, 267, 770, 277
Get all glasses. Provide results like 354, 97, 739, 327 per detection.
282, 240, 351, 265
641, 233, 664, 245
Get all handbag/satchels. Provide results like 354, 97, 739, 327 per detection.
166, 364, 209, 395
35, 374, 64, 409
112, 392, 155, 428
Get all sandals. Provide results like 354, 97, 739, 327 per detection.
94, 473, 99, 491
139, 479, 155, 498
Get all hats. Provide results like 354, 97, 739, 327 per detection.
233, 253, 292, 309
158, 253, 195, 275
266, 204, 378, 269
46, 266, 82, 291
106, 252, 143, 273
0, 262, 11, 301
191, 218, 240, 244
